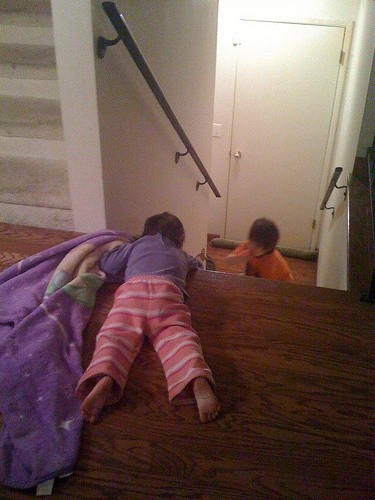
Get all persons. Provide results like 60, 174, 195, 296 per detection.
226, 217, 296, 284
73, 212, 222, 426
